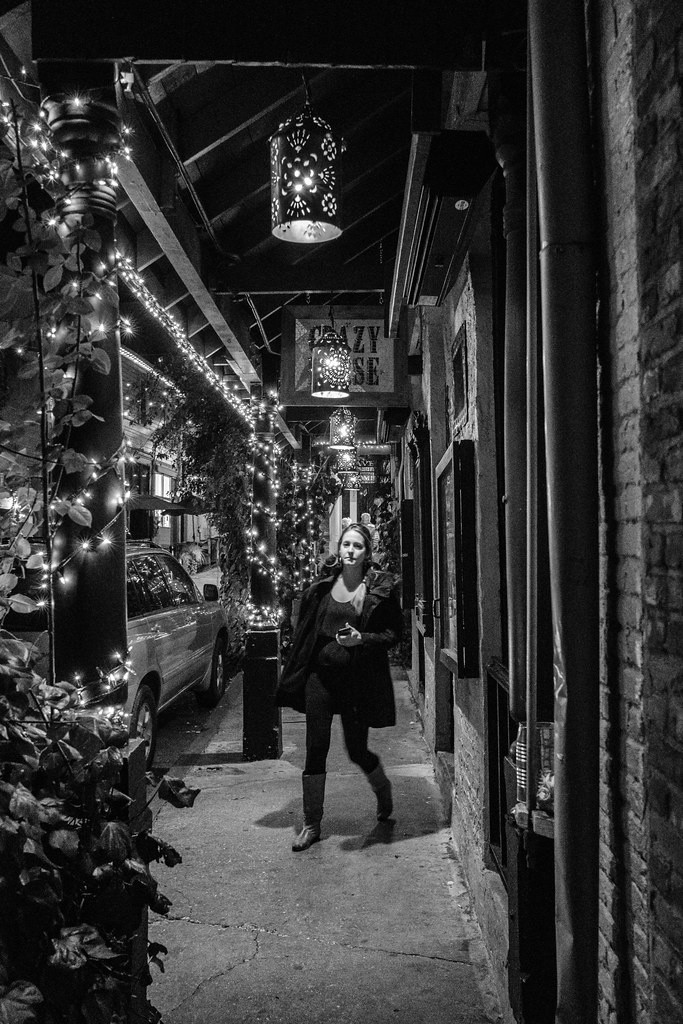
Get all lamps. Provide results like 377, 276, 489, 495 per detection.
266, 72, 345, 243
343, 474, 360, 491
329, 406, 356, 450
310, 294, 351, 399
336, 450, 359, 474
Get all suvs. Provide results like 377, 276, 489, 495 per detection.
124, 540, 231, 772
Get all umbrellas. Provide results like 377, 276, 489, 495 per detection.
128, 493, 187, 539
160, 493, 217, 541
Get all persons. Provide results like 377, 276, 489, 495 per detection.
272, 524, 407, 853
360, 513, 380, 549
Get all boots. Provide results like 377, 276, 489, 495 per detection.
292, 770, 327, 852
364, 762, 393, 822
